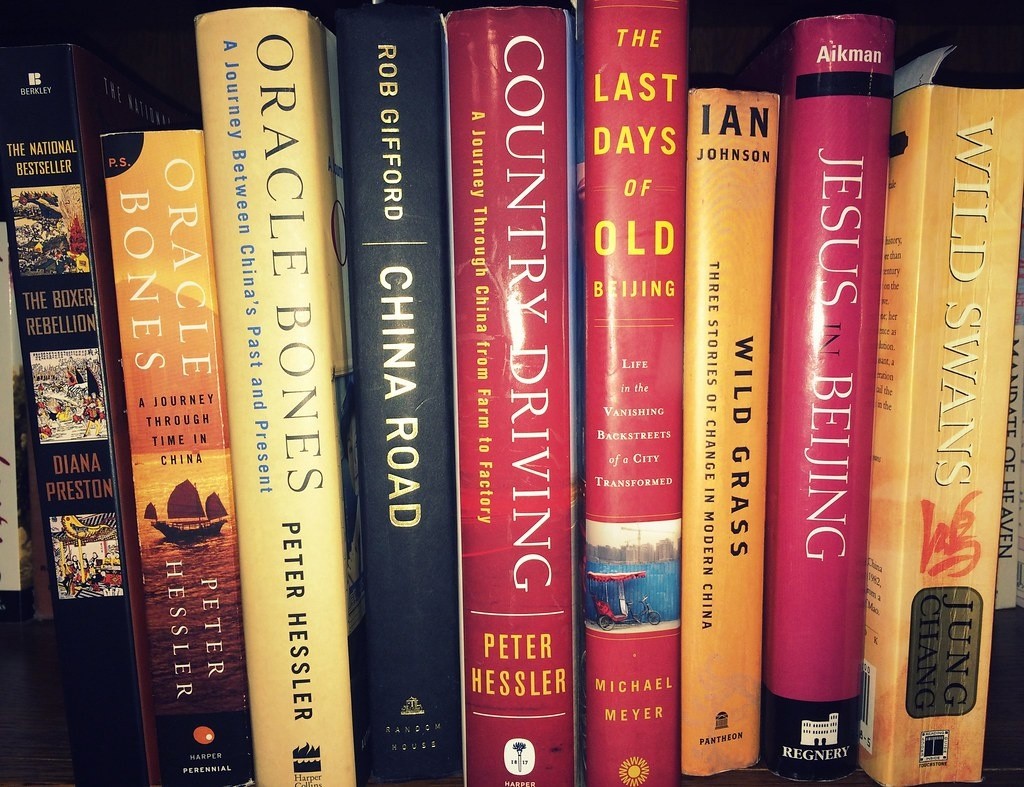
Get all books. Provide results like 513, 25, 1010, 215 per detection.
0, 0, 1024, 787
